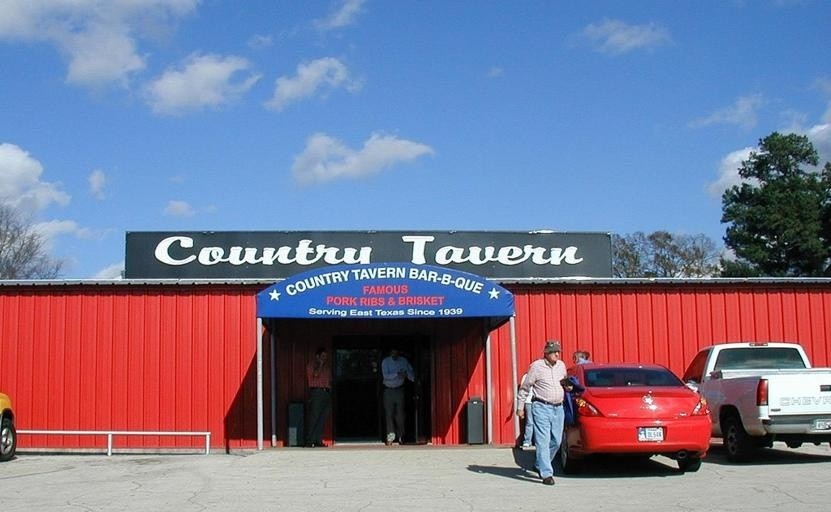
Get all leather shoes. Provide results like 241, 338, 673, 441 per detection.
386, 436, 405, 445
543, 477, 555, 485
303, 441, 325, 447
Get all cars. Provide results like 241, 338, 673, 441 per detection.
0, 393, 17, 461
560, 363, 712, 473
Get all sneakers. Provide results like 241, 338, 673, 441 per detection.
519, 446, 536, 451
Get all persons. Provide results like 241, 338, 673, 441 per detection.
303, 346, 334, 448
382, 346, 416, 446
520, 372, 535, 448
517, 339, 574, 486
572, 350, 593, 365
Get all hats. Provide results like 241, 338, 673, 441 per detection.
544, 341, 561, 353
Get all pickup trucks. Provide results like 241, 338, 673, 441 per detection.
681, 342, 830, 454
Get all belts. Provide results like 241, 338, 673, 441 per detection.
532, 399, 565, 407
386, 384, 404, 390
311, 388, 329, 393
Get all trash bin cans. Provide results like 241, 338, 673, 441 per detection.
467, 397, 483, 445
288, 402, 306, 447
